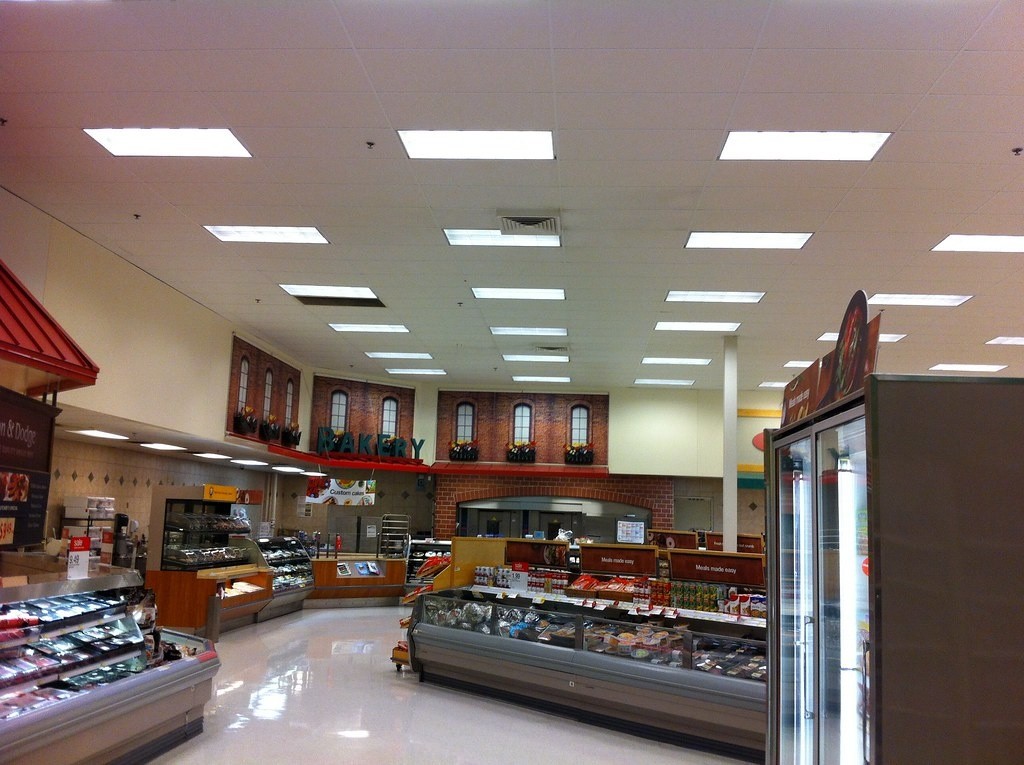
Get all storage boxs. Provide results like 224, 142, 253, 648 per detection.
62, 497, 114, 518
566, 582, 634, 602
65, 526, 114, 565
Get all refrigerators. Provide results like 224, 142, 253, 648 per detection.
764, 374, 1024, 765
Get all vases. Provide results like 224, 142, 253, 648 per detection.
507, 451, 535, 461
449, 449, 478, 461
236, 418, 302, 447
566, 453, 593, 464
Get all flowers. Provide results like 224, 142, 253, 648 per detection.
324, 429, 353, 440
448, 439, 480, 452
505, 441, 538, 454
285, 422, 300, 436
563, 442, 595, 457
385, 436, 405, 447
264, 414, 280, 431
239, 406, 258, 427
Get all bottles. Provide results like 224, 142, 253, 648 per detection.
336, 533, 342, 553
299, 530, 321, 557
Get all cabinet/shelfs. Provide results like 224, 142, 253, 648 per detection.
0, 569, 222, 765
337, 516, 381, 554
145, 484, 273, 644
58, 514, 118, 566
380, 514, 411, 554
390, 537, 506, 672
405, 539, 452, 596
254, 536, 315, 619
